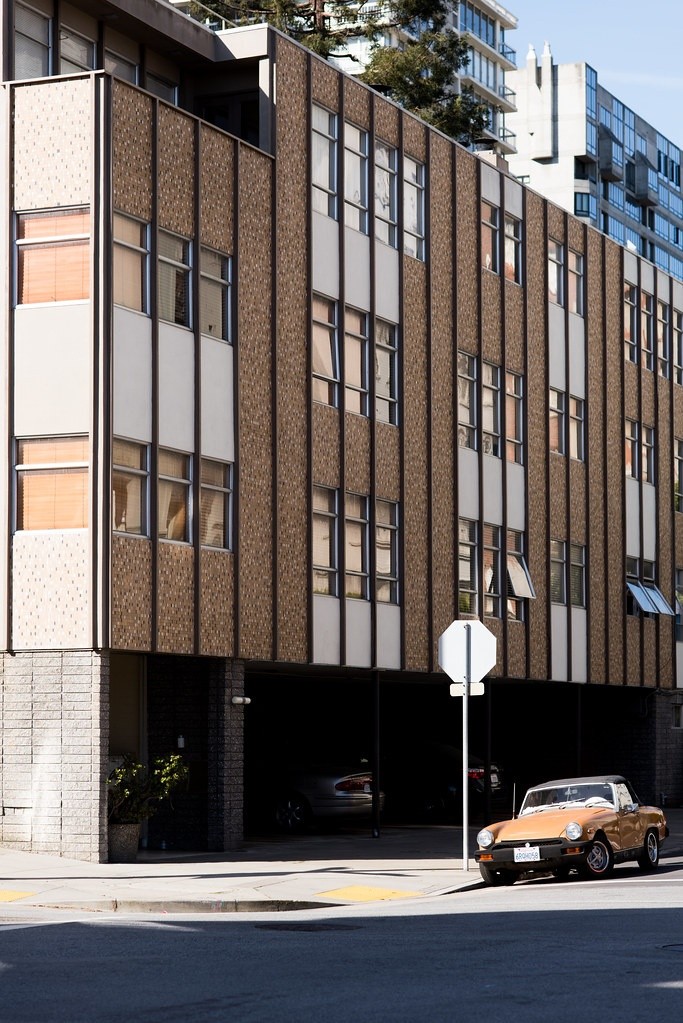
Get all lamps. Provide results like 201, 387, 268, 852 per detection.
232, 695, 252, 706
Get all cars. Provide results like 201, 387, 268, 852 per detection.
265, 747, 387, 838
472, 773, 672, 887
407, 740, 509, 824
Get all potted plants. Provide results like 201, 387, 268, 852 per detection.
108, 751, 190, 863
